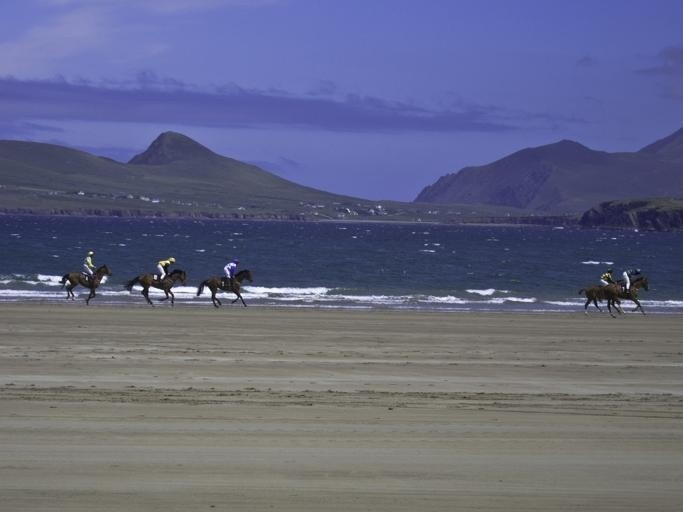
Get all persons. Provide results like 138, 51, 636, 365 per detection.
155, 257, 175, 284
621, 267, 641, 295
81, 249, 96, 282
223, 258, 239, 291
598, 268, 615, 289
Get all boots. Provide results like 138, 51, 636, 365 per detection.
90, 274, 94, 279
626, 289, 631, 293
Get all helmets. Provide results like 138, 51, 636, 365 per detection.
608, 268, 612, 272
170, 258, 175, 262
636, 269, 640, 273
233, 259, 240, 263
88, 252, 94, 256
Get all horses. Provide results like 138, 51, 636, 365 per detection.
578, 276, 650, 318
197, 269, 253, 308
124, 269, 186, 306
59, 262, 112, 306
